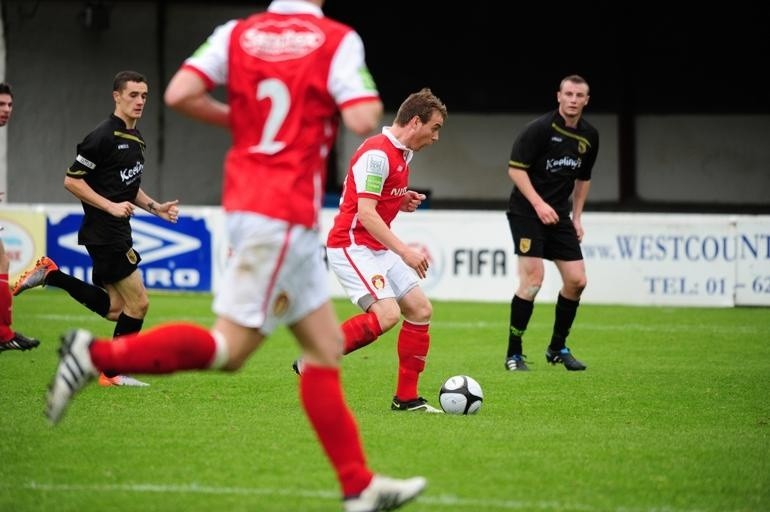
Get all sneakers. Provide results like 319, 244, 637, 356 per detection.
0, 331, 40, 350
292, 358, 307, 376
340, 474, 425, 512
545, 346, 586, 371
392, 396, 444, 413
505, 357, 529, 371
12, 256, 57, 297
99, 372, 150, 387
44, 328, 99, 426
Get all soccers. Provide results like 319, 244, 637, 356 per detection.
439, 374, 483, 415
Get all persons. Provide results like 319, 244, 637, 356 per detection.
289, 87, 450, 415
504, 73, 600, 374
44, 0, 432, 512
0, 82, 41, 353
12, 69, 179, 389
